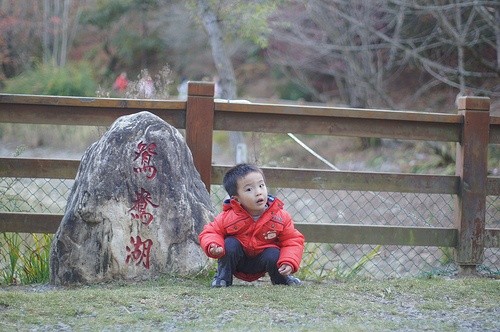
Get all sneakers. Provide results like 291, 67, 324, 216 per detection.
271, 276, 302, 286
211, 275, 232, 287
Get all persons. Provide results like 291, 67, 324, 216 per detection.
199, 162, 305, 287
